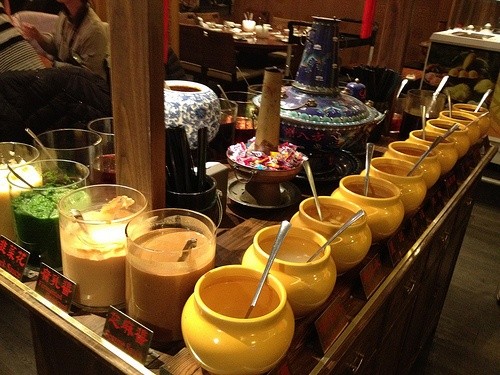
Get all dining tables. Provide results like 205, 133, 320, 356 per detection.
200, 22, 306, 70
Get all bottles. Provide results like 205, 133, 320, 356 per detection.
181, 265, 294, 375
292, 195, 373, 276
241, 224, 338, 321
163, 79, 221, 148
381, 141, 441, 190
329, 167, 404, 245
360, 158, 427, 222
405, 103, 493, 177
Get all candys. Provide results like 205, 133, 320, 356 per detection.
227, 138, 303, 171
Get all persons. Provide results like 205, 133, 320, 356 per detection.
0, 0, 46, 70
20, 0, 112, 80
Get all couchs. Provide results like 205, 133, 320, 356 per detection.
9, 10, 113, 75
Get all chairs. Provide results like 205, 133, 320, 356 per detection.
179, 14, 313, 91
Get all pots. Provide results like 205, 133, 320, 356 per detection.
253, 16, 388, 153
226, 66, 313, 184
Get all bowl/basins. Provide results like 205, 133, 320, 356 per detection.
204, 19, 299, 42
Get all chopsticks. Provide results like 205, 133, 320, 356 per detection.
162, 124, 208, 193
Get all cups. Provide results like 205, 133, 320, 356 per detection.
88, 117, 116, 185
388, 88, 447, 141
35, 129, 103, 186
0, 141, 42, 243
125, 176, 217, 344
59, 184, 148, 314
7, 158, 90, 275
210, 84, 263, 162
